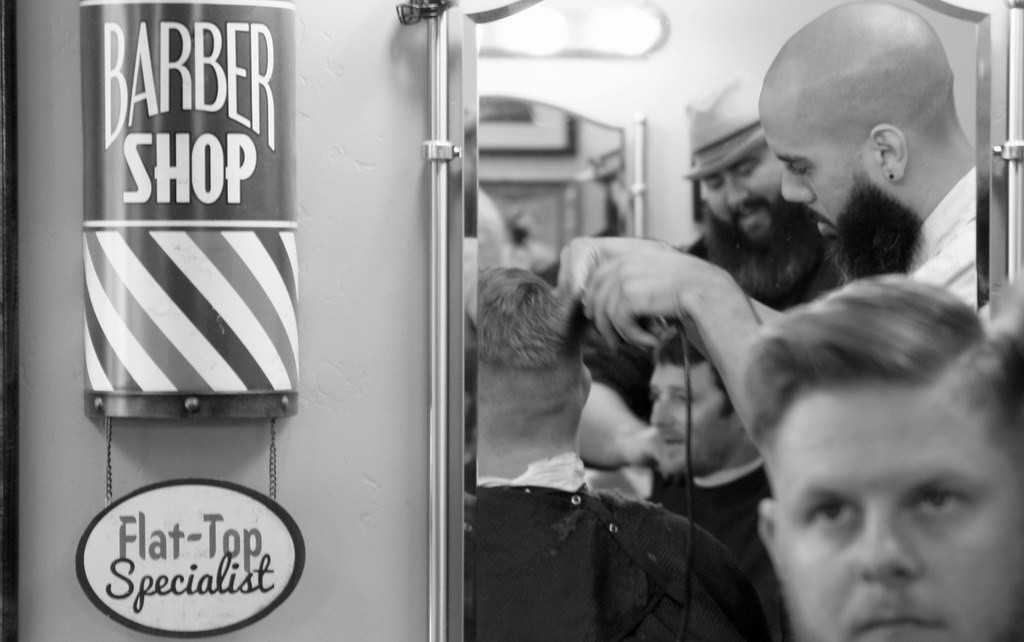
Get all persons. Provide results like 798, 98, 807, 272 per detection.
642, 326, 792, 642
466, 269, 780, 637
572, 77, 841, 497
746, 266, 1024, 642
572, 0, 981, 437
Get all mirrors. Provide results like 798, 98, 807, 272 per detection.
420, 0, 1016, 641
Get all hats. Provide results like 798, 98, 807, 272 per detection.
680, 67, 764, 181
